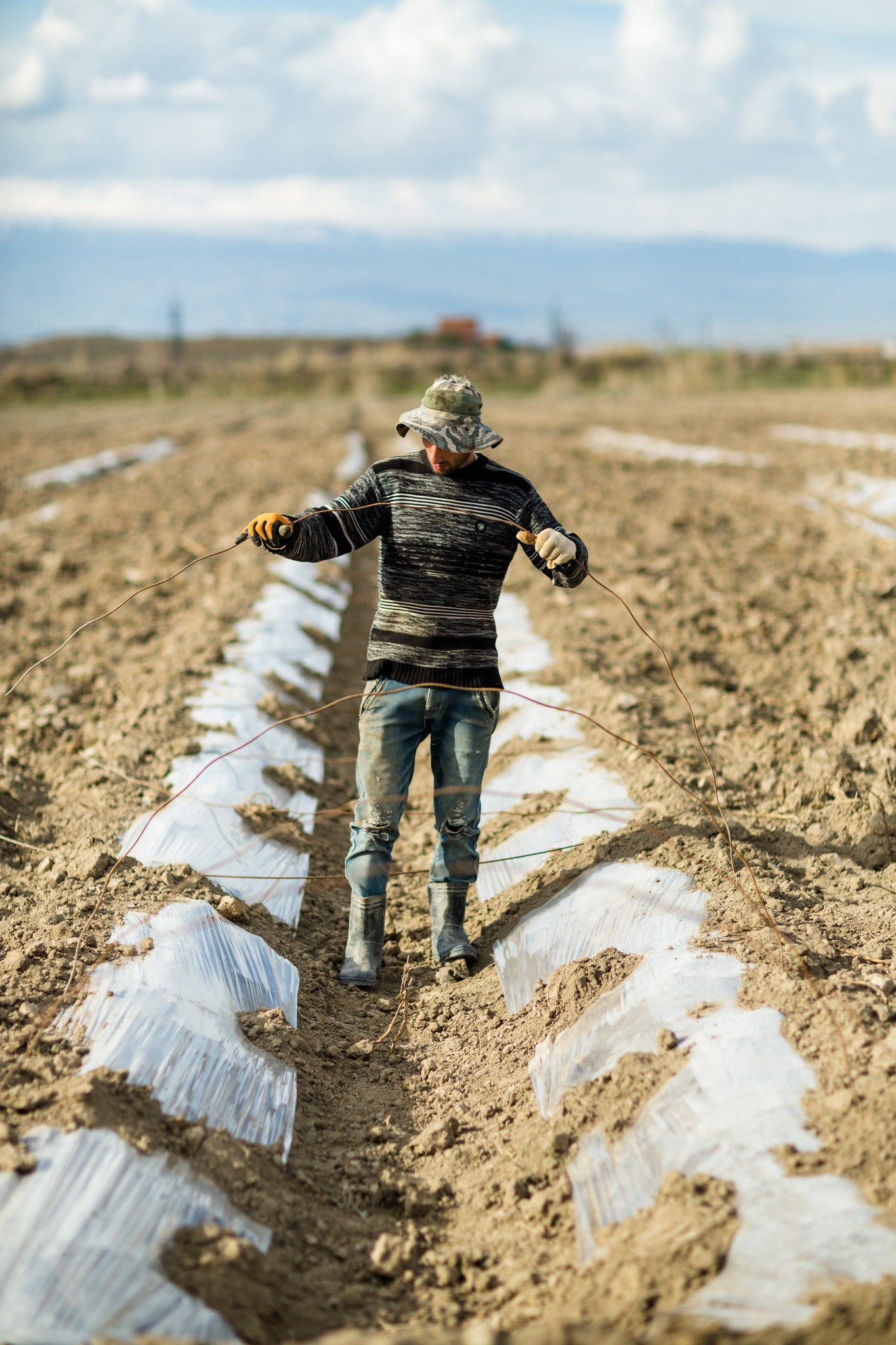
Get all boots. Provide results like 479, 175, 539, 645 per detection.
427, 881, 478, 965
336, 892, 387, 986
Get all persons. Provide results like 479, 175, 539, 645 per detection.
235, 372, 588, 989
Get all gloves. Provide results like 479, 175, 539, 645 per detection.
235, 513, 294, 549
516, 528, 577, 570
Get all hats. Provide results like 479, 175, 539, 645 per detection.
396, 374, 504, 453
